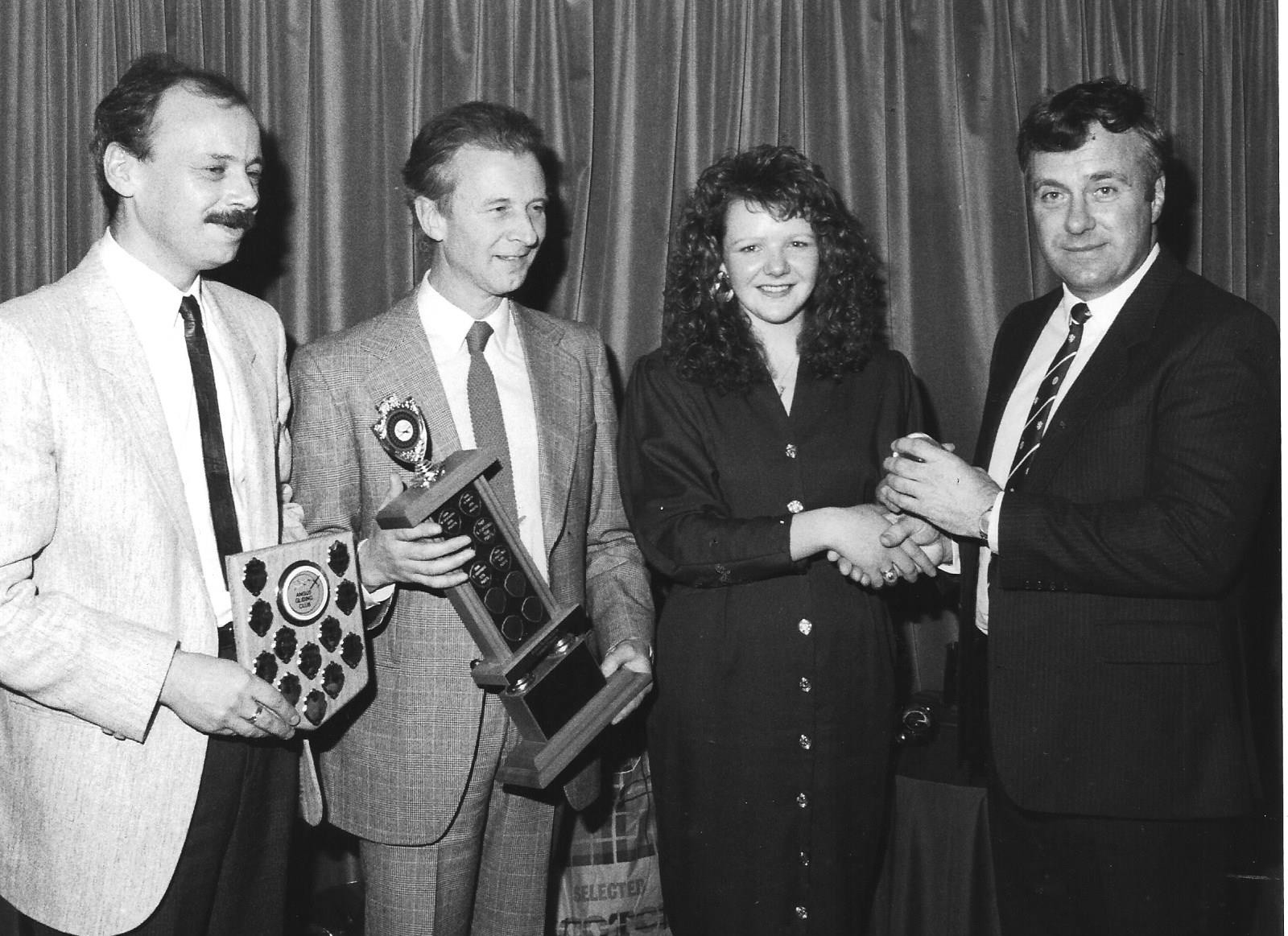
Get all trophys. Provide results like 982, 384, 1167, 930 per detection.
368, 392, 653, 790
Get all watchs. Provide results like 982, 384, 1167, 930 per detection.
979, 505, 994, 540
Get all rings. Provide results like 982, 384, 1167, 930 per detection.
881, 568, 897, 584
247, 704, 263, 723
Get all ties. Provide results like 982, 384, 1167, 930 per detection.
1001, 301, 1093, 495
465, 324, 518, 533
179, 294, 244, 591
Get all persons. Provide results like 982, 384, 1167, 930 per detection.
289, 102, 656, 936
828, 77, 1284, 936
0, 50, 309, 935
618, 144, 955, 935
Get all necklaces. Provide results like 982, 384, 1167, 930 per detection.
776, 353, 798, 397
